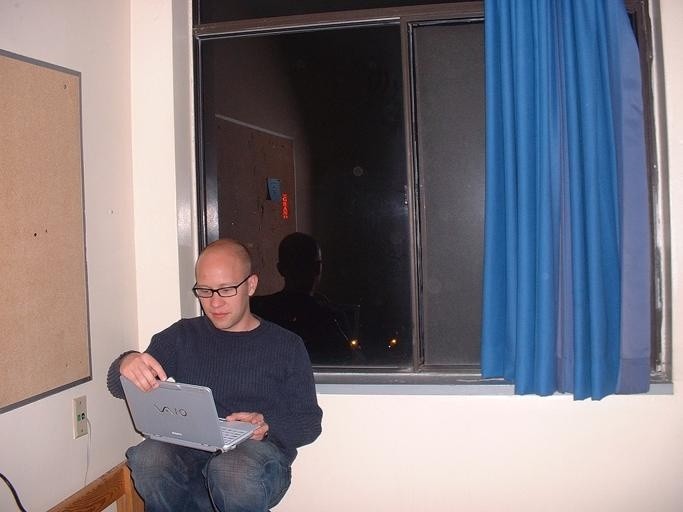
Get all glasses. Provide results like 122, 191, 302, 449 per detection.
192, 276, 250, 297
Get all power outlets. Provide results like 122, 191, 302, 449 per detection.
71, 395, 91, 438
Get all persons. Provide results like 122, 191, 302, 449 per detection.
249, 232, 353, 366
107, 239, 323, 512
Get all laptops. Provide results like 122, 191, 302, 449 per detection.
119, 374, 262, 455
